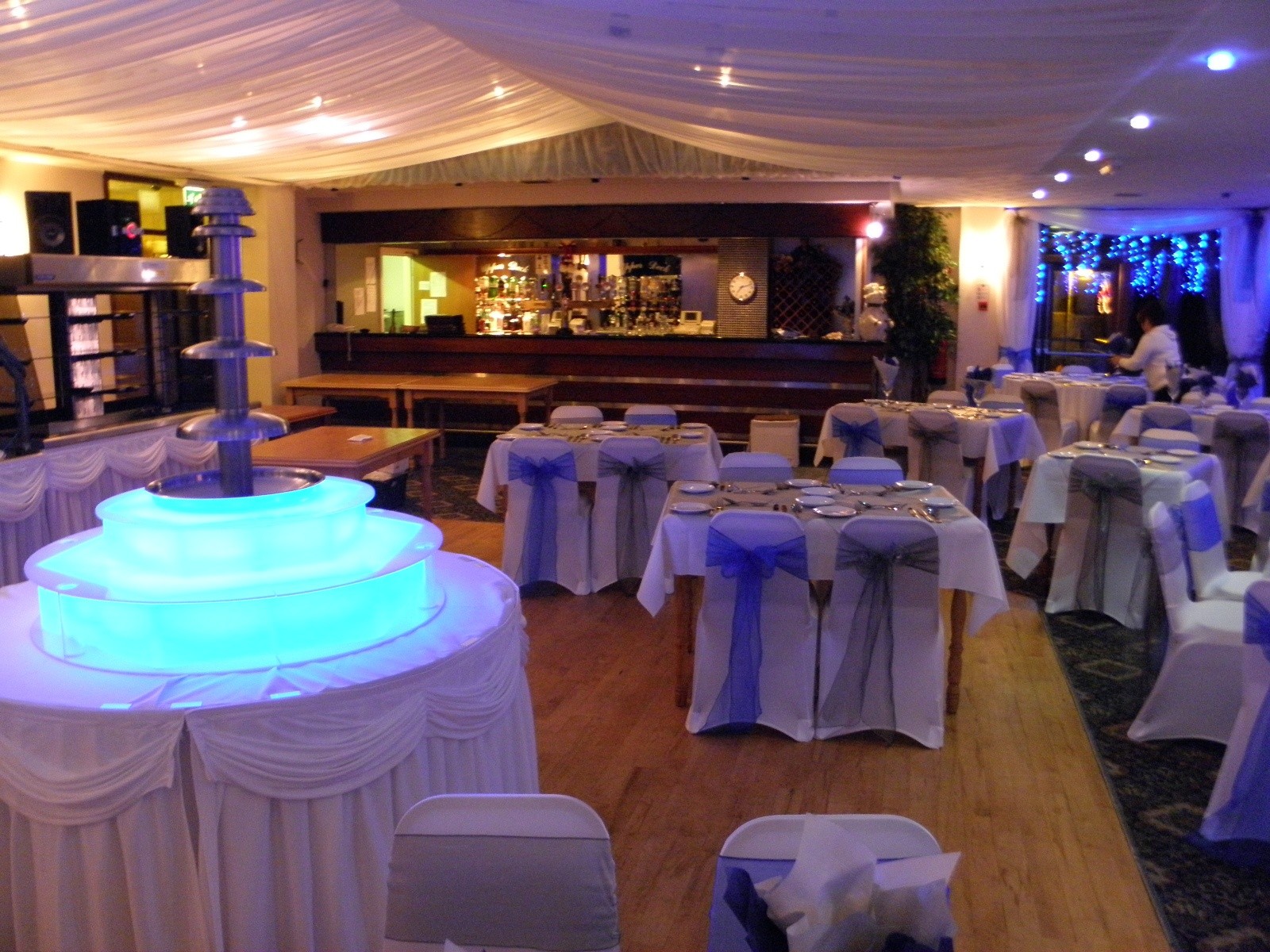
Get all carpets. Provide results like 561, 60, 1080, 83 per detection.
1032, 551, 1270, 952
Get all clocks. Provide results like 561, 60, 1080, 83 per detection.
728, 274, 757, 302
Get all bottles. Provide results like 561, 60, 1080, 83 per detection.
475, 274, 680, 335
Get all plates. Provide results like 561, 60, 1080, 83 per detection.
1002, 369, 1147, 388
1046, 439, 1199, 465
918, 495, 957, 507
802, 487, 839, 497
863, 395, 1024, 422
496, 433, 525, 440
1133, 400, 1270, 418
518, 423, 543, 430
784, 478, 822, 487
795, 495, 835, 506
813, 505, 857, 516
669, 501, 712, 515
678, 483, 715, 494
895, 480, 933, 490
679, 422, 708, 439
588, 421, 628, 441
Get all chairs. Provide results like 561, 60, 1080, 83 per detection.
381, 344, 1270, 952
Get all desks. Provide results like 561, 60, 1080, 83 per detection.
813, 400, 1045, 527
1109, 404, 1270, 454
1004, 441, 1232, 580
636, 480, 1011, 716
0, 370, 560, 951
1004, 373, 1155, 441
477, 422, 723, 513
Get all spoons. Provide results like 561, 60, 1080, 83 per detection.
722, 497, 769, 505
859, 500, 896, 507
908, 508, 920, 519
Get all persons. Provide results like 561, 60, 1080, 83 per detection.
1108, 305, 1183, 403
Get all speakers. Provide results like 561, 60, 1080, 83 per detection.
77, 199, 144, 258
165, 204, 208, 260
25, 191, 75, 256
